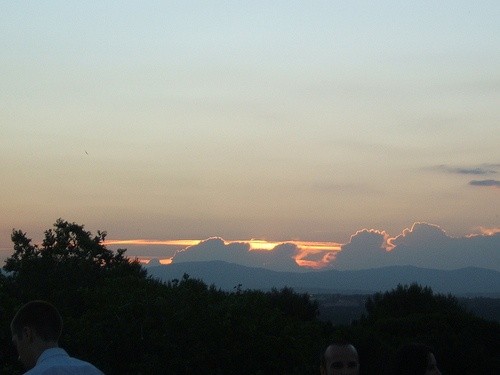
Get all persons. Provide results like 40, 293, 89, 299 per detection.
320, 340, 361, 375
11, 301, 103, 374
393, 342, 441, 375
